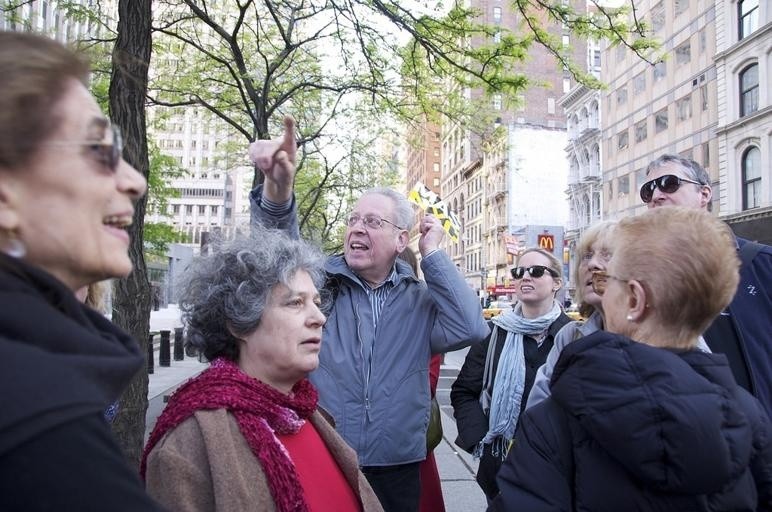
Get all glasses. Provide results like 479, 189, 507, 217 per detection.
510, 265, 559, 280
10, 124, 122, 173
591, 270, 628, 297
348, 215, 404, 230
639, 174, 701, 203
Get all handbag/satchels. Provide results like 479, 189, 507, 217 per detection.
426, 398, 443, 453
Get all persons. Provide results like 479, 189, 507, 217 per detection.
139, 209, 385, 512
640, 154, 772, 422
486, 205, 772, 512
525, 219, 712, 411
397, 247, 445, 512
450, 248, 575, 505
0, 30, 174, 512
74, 280, 119, 424
248, 115, 492, 512
